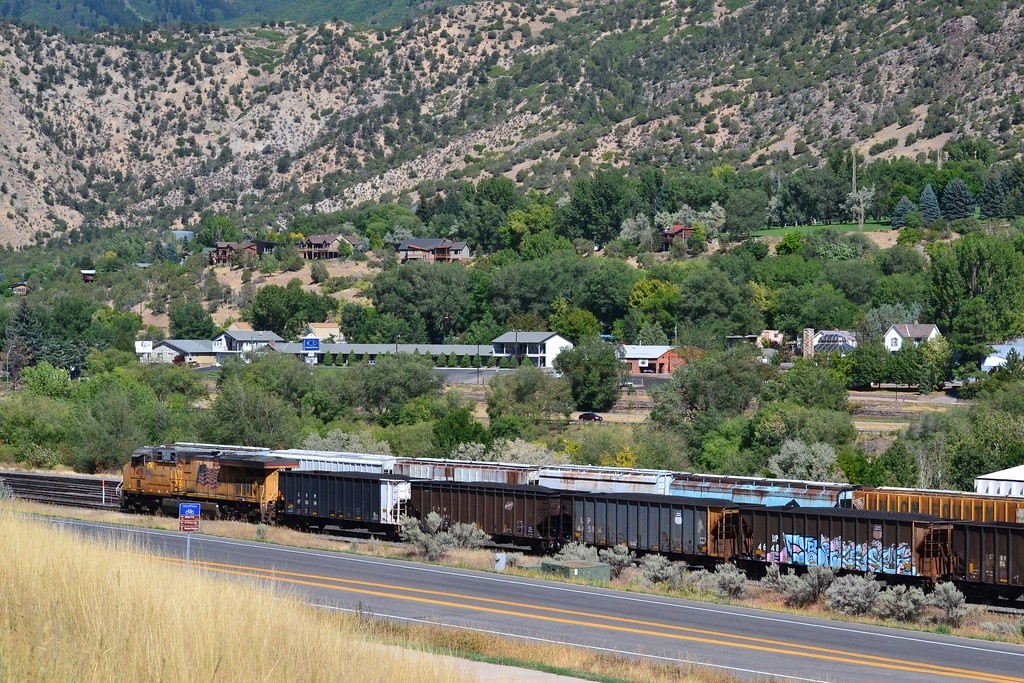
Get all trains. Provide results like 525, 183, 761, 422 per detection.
171, 440, 1023, 524
115, 444, 1023, 606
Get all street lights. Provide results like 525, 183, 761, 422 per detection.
6, 346, 17, 388
512, 328, 521, 356
226, 298, 234, 318
396, 334, 400, 361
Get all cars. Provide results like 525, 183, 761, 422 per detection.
578, 413, 603, 421
